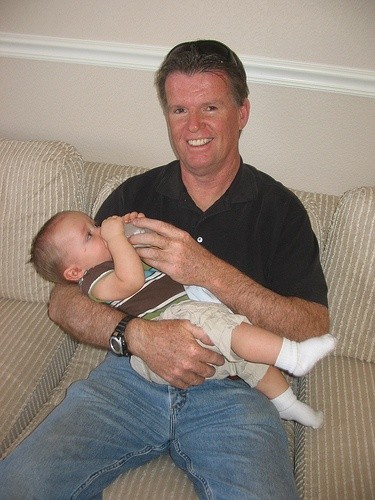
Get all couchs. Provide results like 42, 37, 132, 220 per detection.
0, 137, 375, 500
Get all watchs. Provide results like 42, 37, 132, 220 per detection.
109, 314, 135, 356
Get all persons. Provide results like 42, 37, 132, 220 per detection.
0, 39, 330, 500
30, 210, 337, 428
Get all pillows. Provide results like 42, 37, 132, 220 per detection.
90, 175, 324, 262
320, 185, 375, 365
0, 139, 87, 303
